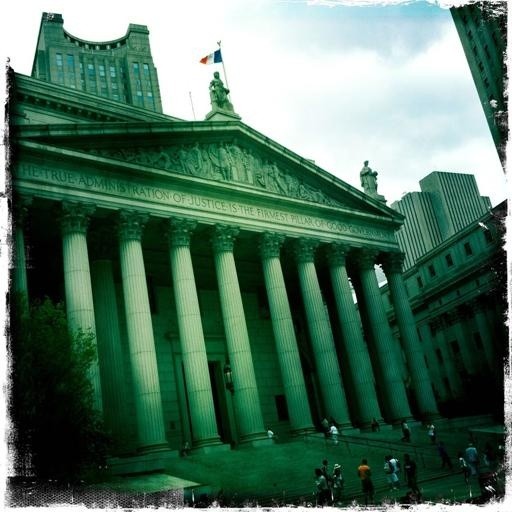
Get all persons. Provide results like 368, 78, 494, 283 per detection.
267, 417, 339, 445
208, 71, 233, 112
359, 160, 378, 196
371, 418, 505, 502
315, 459, 375, 505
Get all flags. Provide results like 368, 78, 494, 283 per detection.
199, 47, 222, 64
